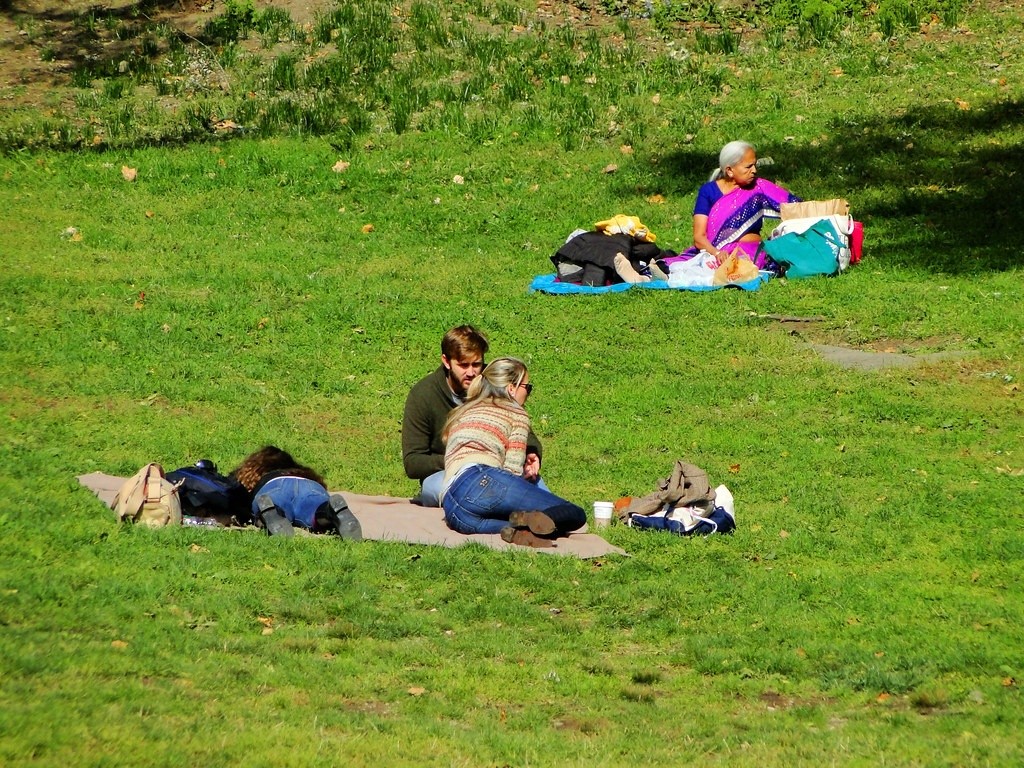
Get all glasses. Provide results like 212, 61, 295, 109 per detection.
512, 383, 533, 394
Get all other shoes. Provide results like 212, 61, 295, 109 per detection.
256, 494, 295, 541
507, 511, 556, 535
324, 494, 362, 542
499, 526, 552, 549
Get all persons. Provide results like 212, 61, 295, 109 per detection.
402, 327, 553, 504
442, 358, 587, 547
614, 139, 803, 287
227, 447, 361, 543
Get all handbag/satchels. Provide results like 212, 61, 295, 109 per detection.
122, 463, 182, 529
764, 198, 864, 283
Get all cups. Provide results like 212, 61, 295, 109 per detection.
593, 502, 614, 529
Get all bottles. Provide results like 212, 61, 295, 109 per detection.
182, 515, 217, 529
847, 222, 863, 264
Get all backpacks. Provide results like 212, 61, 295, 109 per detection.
629, 483, 736, 537
163, 465, 250, 518
